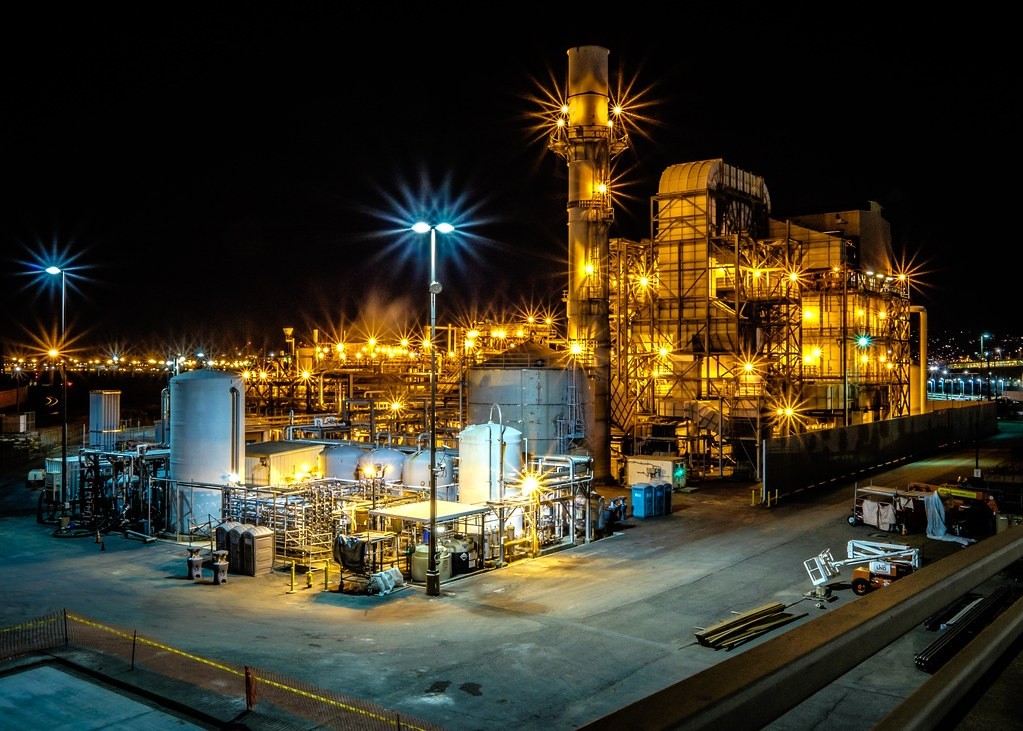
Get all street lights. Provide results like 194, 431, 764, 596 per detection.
412, 222, 458, 596
45, 264, 68, 506
927, 374, 1007, 397
980, 334, 989, 401
